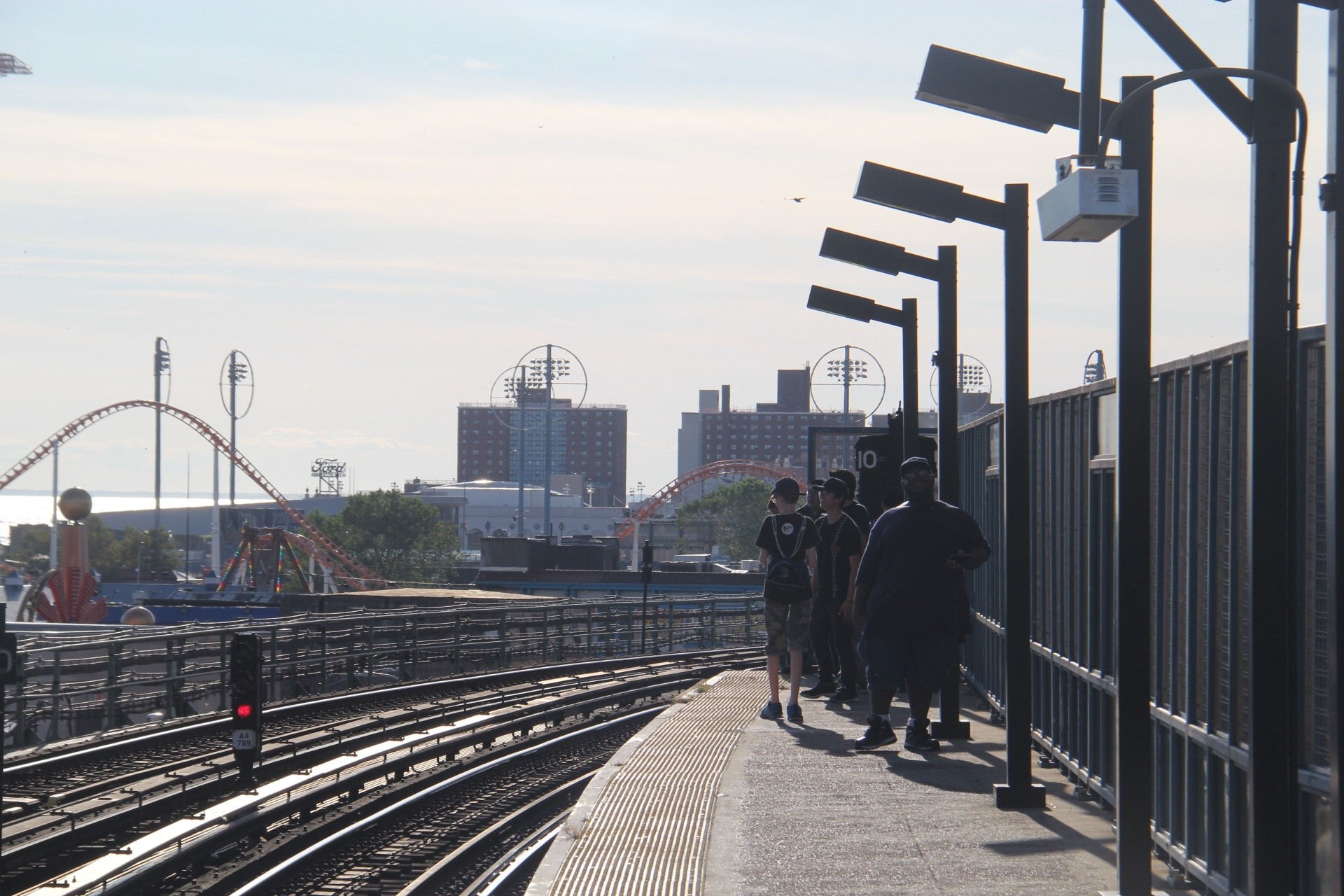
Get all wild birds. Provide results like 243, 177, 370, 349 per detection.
786, 197, 807, 203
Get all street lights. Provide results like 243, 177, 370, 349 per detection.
913, 41, 1160, 896
154, 335, 172, 585
219, 347, 255, 507
511, 343, 588, 537
849, 155, 1049, 813
816, 220, 973, 746
809, 344, 887, 469
807, 282, 921, 504
489, 365, 556, 538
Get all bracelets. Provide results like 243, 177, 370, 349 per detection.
846, 599, 853, 603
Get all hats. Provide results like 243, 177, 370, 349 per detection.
899, 456, 932, 476
769, 477, 800, 495
813, 477, 848, 495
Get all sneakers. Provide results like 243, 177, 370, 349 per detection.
759, 701, 784, 719
903, 718, 939, 751
829, 686, 859, 703
799, 681, 838, 699
786, 699, 803, 722
854, 714, 898, 750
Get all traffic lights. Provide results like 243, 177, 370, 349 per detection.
230, 633, 262, 728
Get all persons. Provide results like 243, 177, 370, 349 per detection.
755, 457, 992, 751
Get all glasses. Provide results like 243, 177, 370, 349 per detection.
771, 494, 778, 503
902, 469, 931, 480
818, 489, 835, 495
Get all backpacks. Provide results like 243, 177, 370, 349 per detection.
762, 514, 807, 604
